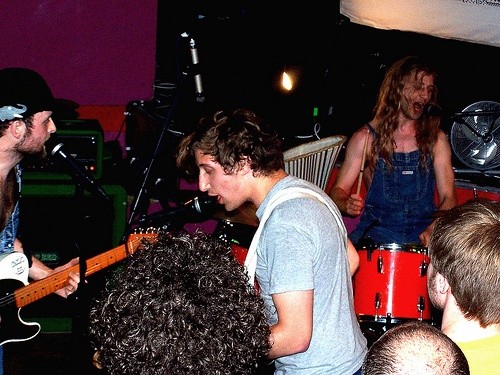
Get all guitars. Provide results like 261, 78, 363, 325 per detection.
0, 225, 169, 345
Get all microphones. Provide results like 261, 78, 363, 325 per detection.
422, 103, 465, 125
45, 138, 112, 206
135, 195, 217, 227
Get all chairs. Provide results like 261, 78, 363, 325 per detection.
282, 134, 348, 192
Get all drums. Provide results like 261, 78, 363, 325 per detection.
353, 243, 433, 334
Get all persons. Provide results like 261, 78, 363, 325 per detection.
0, 67, 88, 375
324, 55, 457, 245
363, 320, 469, 375
92, 225, 275, 375
428, 198, 500, 374
176, 107, 370, 375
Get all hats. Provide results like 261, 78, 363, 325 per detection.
0, 68, 56, 123
